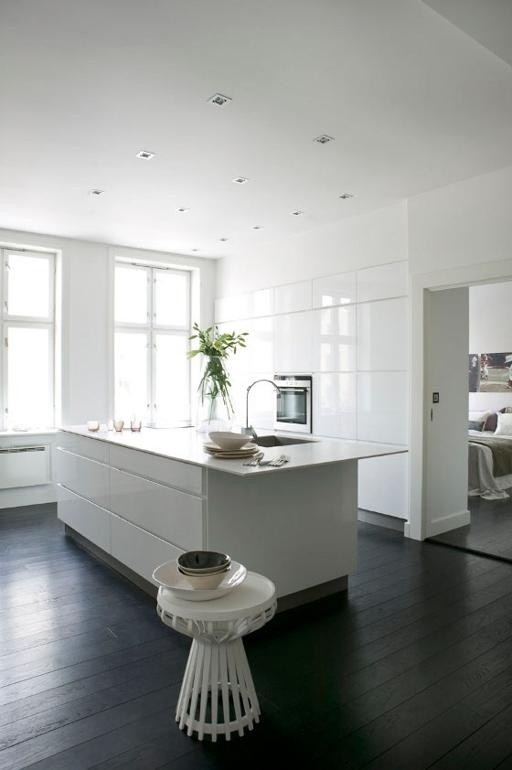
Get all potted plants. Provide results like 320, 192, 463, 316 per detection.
189, 322, 249, 432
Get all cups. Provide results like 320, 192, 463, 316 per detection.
130, 420, 142, 433
86, 420, 103, 432
112, 419, 124, 432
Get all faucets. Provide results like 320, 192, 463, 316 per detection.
241, 379, 282, 438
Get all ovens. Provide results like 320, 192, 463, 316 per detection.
271, 372, 312, 434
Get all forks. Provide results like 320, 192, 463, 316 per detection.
242, 448, 293, 468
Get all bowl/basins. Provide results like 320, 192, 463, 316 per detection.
174, 550, 233, 589
208, 428, 255, 450
152, 559, 246, 603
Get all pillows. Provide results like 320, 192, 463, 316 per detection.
469, 411, 492, 433
493, 410, 512, 438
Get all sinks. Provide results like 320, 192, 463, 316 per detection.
249, 434, 320, 447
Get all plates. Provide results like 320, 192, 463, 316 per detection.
201, 445, 260, 460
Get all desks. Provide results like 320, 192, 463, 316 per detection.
56, 421, 413, 633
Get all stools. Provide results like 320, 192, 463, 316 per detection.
153, 571, 277, 743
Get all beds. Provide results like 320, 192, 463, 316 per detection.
470, 407, 512, 502
